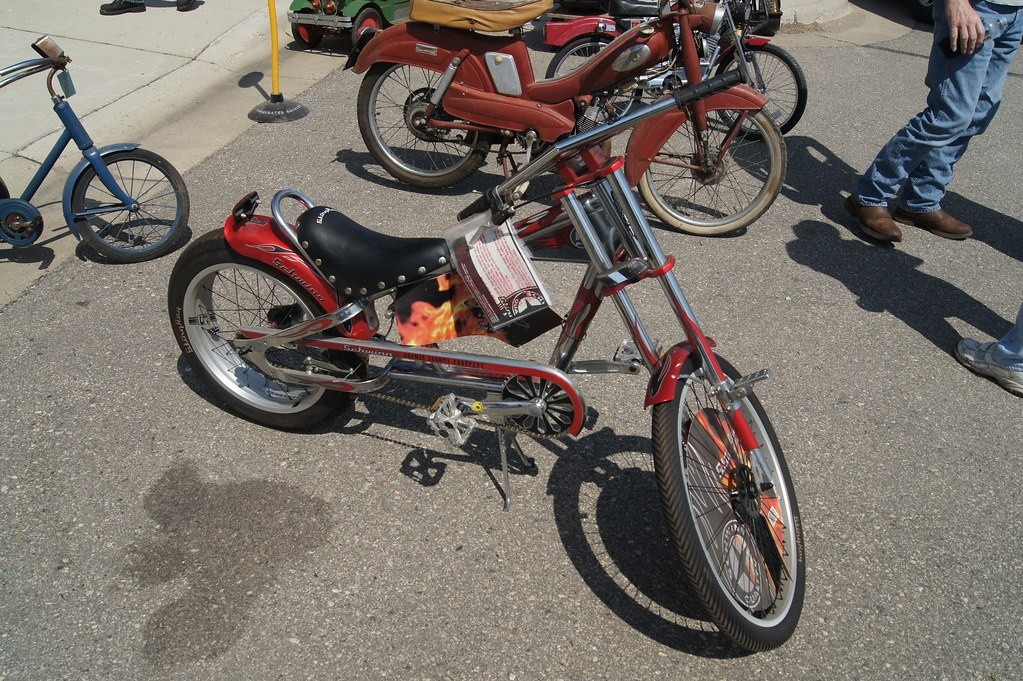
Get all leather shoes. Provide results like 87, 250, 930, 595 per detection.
893, 202, 973, 240
176, 0, 196, 11
100, 0, 146, 15
844, 194, 903, 242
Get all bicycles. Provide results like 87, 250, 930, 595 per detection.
173, 65, 808, 655
1, 36, 190, 264
289, 1, 810, 239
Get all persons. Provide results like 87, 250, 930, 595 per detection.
843, 0, 1023, 244
100, 0, 195, 15
954, 301, 1023, 398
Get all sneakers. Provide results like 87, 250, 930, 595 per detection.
954, 338, 1023, 394
